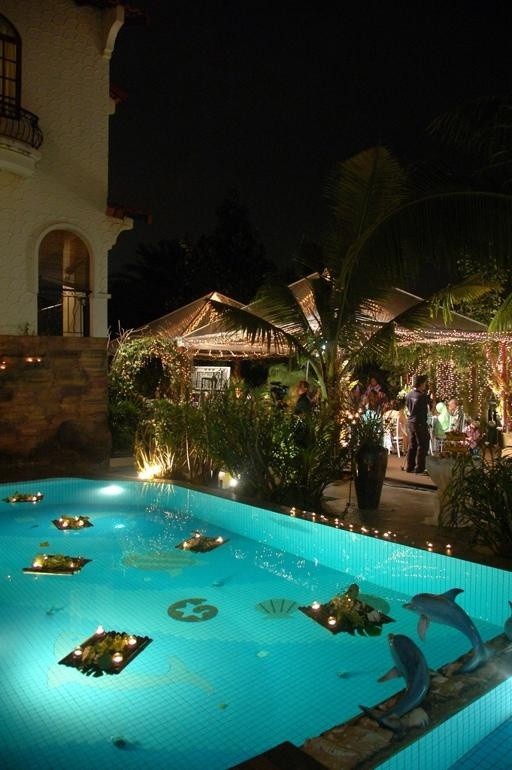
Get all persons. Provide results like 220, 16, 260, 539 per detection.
365, 378, 386, 418
405, 375, 433, 473
292, 381, 312, 416
436, 399, 463, 436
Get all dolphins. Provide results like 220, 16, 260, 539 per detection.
402, 588, 498, 678
357, 632, 437, 735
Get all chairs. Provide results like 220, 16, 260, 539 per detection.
426, 415, 443, 456
390, 415, 404, 457
